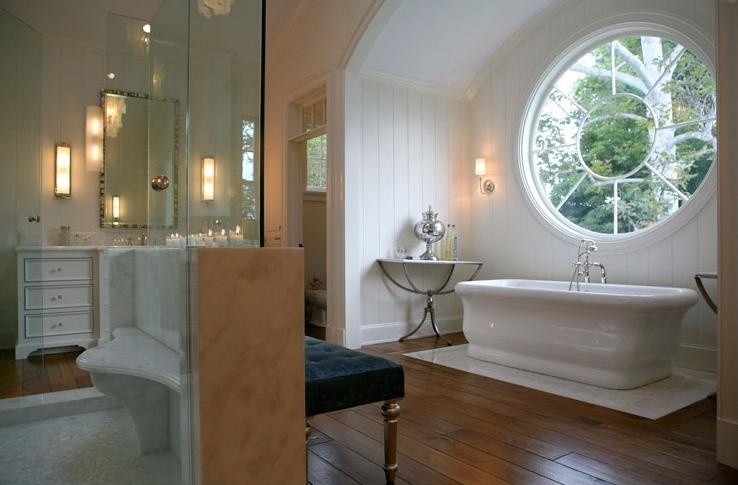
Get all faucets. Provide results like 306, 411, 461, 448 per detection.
314, 276, 323, 284
139, 231, 146, 247
573, 262, 590, 278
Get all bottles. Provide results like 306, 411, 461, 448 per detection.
58, 224, 70, 246
443, 223, 460, 261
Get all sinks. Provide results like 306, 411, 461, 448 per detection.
305, 289, 327, 311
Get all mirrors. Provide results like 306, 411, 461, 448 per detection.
99, 86, 181, 230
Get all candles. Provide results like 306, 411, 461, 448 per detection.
165, 233, 246, 249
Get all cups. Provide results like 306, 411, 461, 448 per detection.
397, 242, 409, 258
165, 229, 245, 247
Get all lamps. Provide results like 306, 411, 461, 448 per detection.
475, 156, 496, 195
53, 142, 72, 198
111, 193, 121, 220
200, 155, 216, 204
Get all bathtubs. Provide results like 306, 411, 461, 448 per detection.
453, 279, 699, 392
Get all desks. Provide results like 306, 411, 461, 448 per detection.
378, 259, 483, 347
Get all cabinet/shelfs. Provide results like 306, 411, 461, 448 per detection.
14, 244, 101, 366
